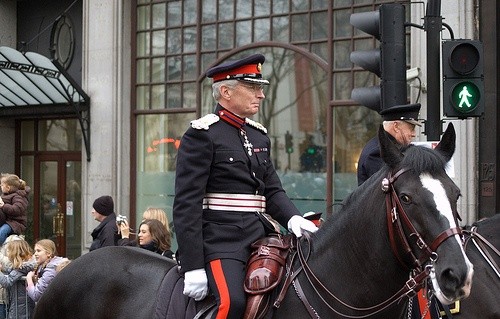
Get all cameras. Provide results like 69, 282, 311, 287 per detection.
116, 215, 127, 225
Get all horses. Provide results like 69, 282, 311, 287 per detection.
409, 214, 499, 319
30, 121, 474, 319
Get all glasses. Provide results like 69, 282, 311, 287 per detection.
236, 83, 264, 90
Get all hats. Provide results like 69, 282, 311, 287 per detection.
93, 196, 114, 216
207, 54, 270, 86
380, 103, 422, 127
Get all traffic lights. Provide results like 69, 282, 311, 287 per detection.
284, 133, 293, 153
300, 138, 326, 168
440, 40, 485, 117
349, 3, 407, 118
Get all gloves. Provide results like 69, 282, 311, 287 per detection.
183, 268, 207, 301
288, 215, 319, 237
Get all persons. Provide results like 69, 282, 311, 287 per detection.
0, 173, 31, 246
0, 239, 36, 319
357, 102, 422, 186
138, 218, 174, 261
89, 195, 121, 253
173, 53, 322, 319
115, 208, 170, 248
25, 238, 69, 304
0, 234, 26, 319
